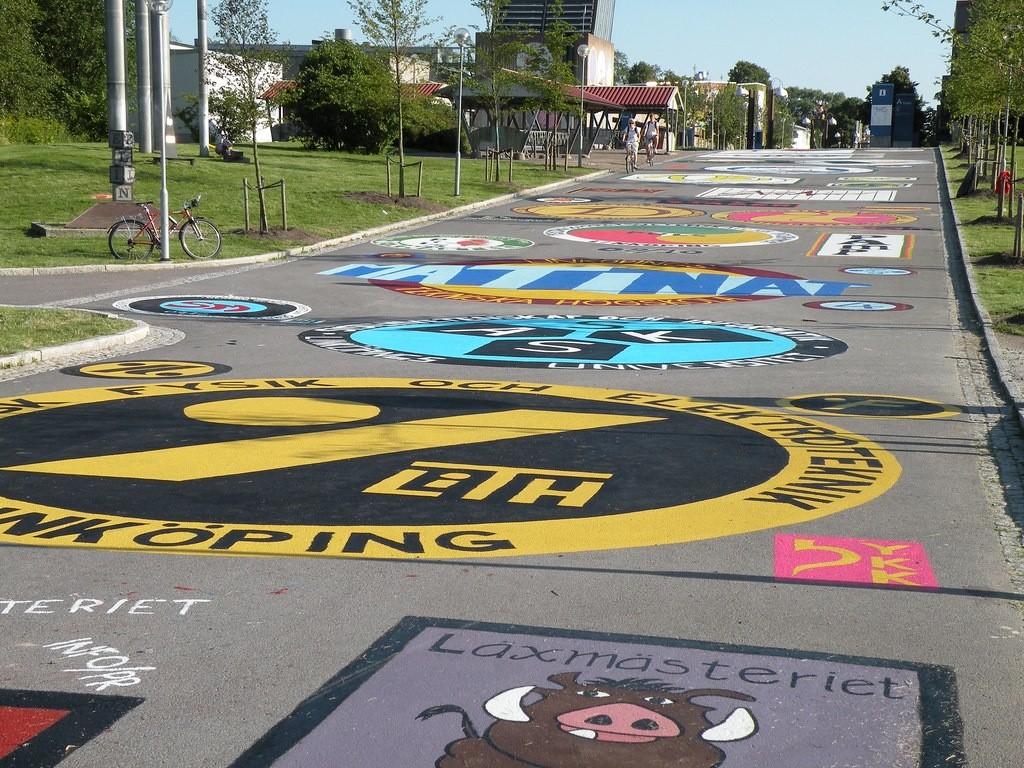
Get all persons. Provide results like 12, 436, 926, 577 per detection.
622, 117, 641, 169
643, 113, 660, 163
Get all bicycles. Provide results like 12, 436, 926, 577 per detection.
106, 195, 223, 262
623, 140, 638, 175
643, 135, 658, 167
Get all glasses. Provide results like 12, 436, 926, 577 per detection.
629, 123, 634, 124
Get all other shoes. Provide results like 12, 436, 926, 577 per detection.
646, 158, 649, 163
654, 149, 657, 153
634, 166, 638, 169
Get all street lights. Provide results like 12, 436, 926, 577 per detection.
709, 89, 717, 150
682, 80, 689, 150
452, 28, 470, 196
146, 0, 170, 263
741, 101, 749, 150
781, 118, 786, 149
577, 44, 591, 167
